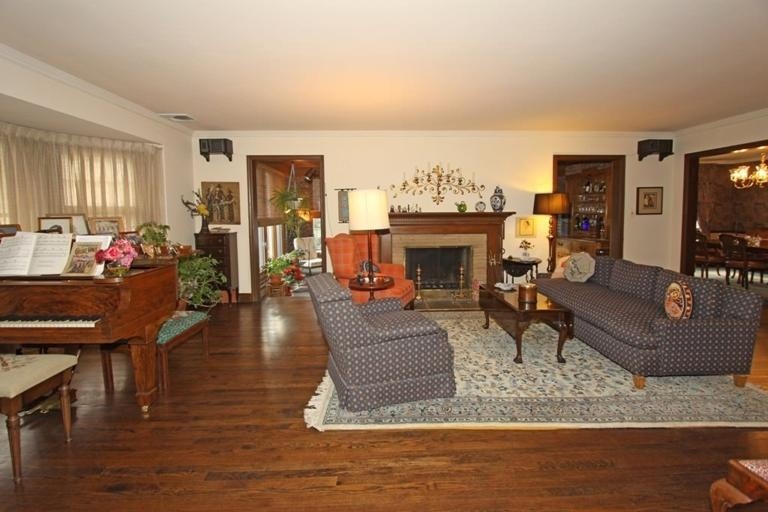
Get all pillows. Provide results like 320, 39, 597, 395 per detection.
563, 251, 596, 282
663, 280, 692, 321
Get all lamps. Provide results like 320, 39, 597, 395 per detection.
348, 189, 390, 282
729, 153, 768, 189
533, 192, 569, 278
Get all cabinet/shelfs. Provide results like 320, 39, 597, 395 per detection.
194, 232, 239, 308
566, 168, 610, 237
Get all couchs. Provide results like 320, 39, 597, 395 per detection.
305, 272, 456, 412
531, 255, 763, 389
324, 234, 416, 310
350, 231, 404, 279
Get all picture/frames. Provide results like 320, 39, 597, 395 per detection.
635, 186, 664, 215
88, 216, 124, 235
201, 181, 241, 225
37, 216, 73, 234
45, 213, 92, 234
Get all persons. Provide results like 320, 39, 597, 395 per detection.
205, 184, 235, 223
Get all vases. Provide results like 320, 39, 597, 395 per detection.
107, 260, 130, 275
286, 284, 293, 296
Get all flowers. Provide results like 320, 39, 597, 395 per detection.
94, 239, 138, 266
283, 260, 305, 284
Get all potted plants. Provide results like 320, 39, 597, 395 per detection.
519, 240, 535, 260
269, 184, 301, 208
262, 256, 289, 282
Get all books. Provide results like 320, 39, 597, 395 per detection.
0, 234, 73, 276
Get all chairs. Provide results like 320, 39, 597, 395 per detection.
693, 231, 722, 279
718, 232, 768, 289
293, 237, 323, 276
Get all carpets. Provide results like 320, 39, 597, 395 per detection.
304, 310, 768, 431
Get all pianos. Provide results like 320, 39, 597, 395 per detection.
0, 258, 179, 413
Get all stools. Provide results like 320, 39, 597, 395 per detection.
0, 352, 79, 486
101, 309, 211, 396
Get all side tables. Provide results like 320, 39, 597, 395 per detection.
349, 276, 394, 301
